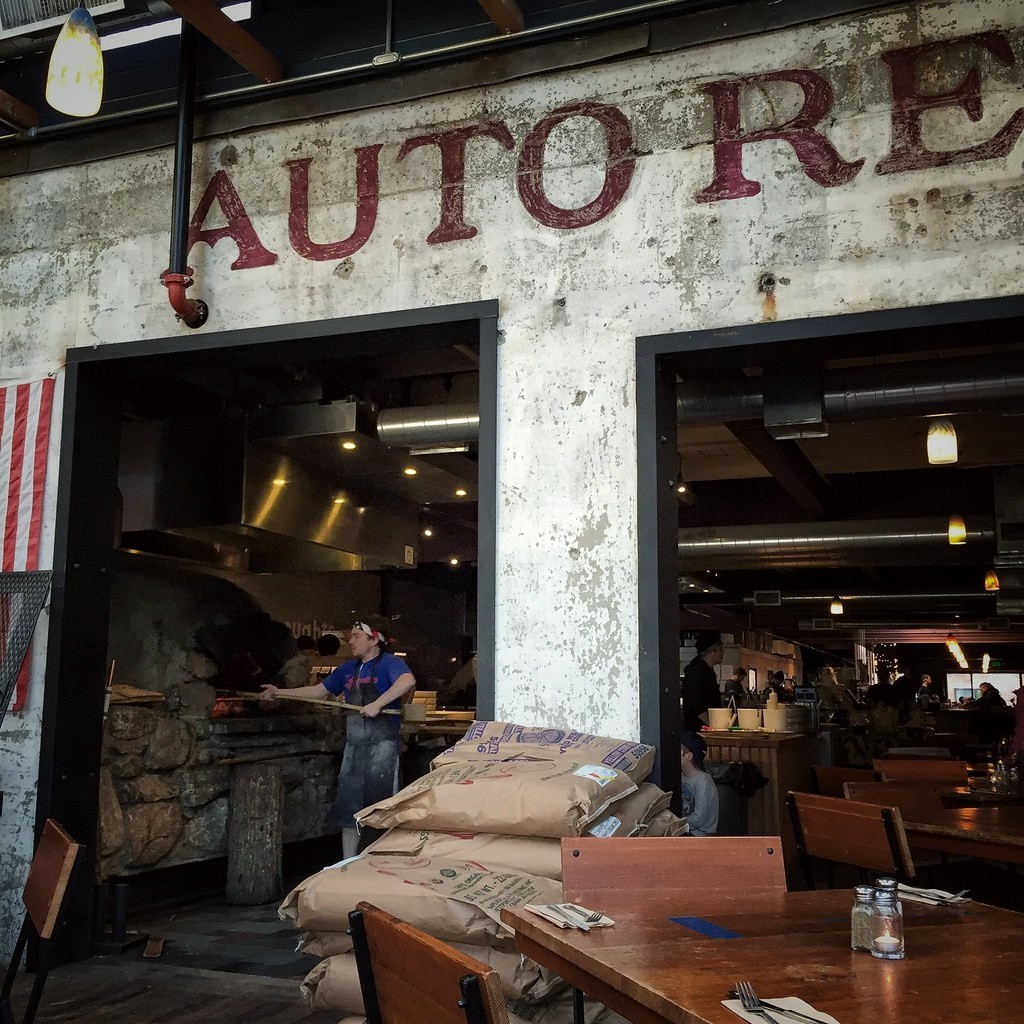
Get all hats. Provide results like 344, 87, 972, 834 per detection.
682, 734, 708, 772
695, 629, 722, 653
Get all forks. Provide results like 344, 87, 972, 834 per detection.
562, 905, 603, 922
907, 890, 970, 900
735, 981, 780, 1024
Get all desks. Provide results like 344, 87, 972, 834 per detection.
898, 805, 1024, 864
501, 889, 1024, 1024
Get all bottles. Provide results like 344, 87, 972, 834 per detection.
769, 688, 778, 709
874, 877, 902, 918
973, 757, 991, 793
995, 764, 1009, 786
851, 884, 873, 951
1008, 768, 1020, 783
747, 690, 763, 701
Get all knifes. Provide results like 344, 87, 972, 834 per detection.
729, 991, 829, 1024
897, 889, 951, 903
547, 904, 590, 930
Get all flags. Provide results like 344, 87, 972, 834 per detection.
0, 378, 55, 710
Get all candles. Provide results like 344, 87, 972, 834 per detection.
875, 930, 900, 952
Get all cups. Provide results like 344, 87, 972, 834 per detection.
767, 700, 771, 709
777, 703, 785, 709
403, 704, 427, 721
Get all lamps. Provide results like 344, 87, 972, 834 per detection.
830, 595, 843, 614
984, 567, 1000, 591
982, 652, 991, 673
409, 444, 469, 456
949, 513, 966, 545
927, 417, 958, 464
678, 454, 686, 493
946, 632, 969, 669
45, 0, 104, 117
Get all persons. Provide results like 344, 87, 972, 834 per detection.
892, 667, 919, 702
724, 667, 745, 709
260, 615, 416, 862
918, 673, 933, 695
683, 632, 722, 732
681, 731, 719, 837
977, 683, 1002, 709
869, 668, 893, 703
278, 634, 340, 688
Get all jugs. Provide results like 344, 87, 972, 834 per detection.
737, 709, 762, 730
708, 708, 737, 728
763, 709, 786, 731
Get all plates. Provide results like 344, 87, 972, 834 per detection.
701, 729, 731, 732
728, 727, 741, 730
763, 731, 794, 733
732, 729, 761, 732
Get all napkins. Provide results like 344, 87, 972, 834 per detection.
524, 902, 616, 933
720, 996, 841, 1024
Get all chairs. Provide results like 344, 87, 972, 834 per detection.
348, 902, 512, 1024
561, 835, 787, 1024
0, 819, 88, 1024
784, 725, 970, 889
